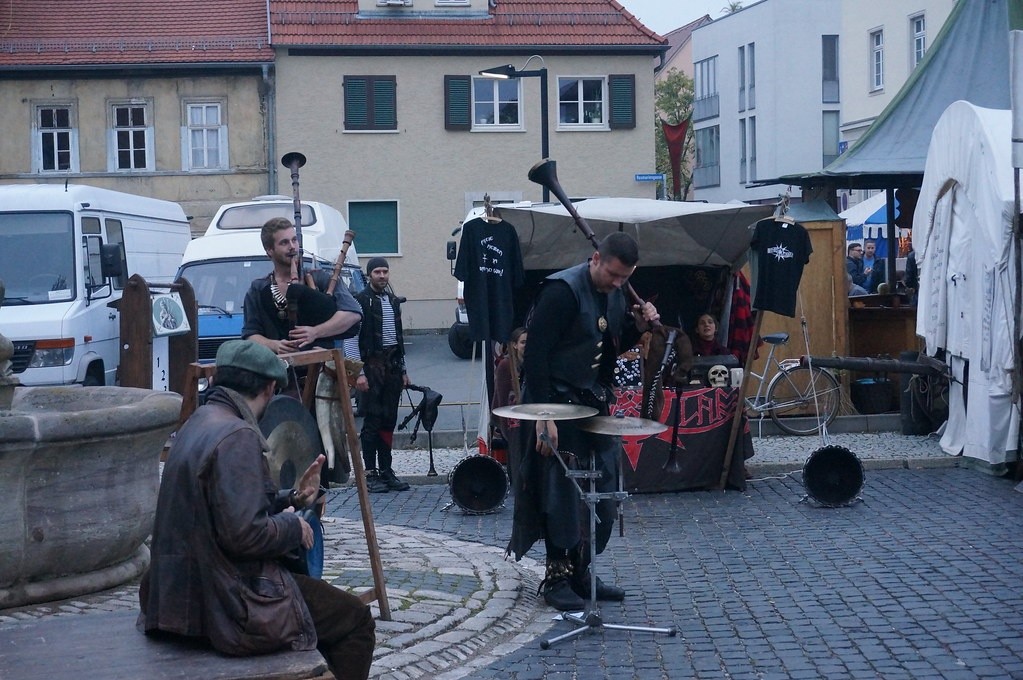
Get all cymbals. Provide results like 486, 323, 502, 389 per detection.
492, 402, 600, 422
576, 414, 669, 437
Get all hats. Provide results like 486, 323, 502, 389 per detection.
367, 257, 389, 276
215, 340, 288, 396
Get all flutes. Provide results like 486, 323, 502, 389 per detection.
398, 381, 445, 476
270, 150, 356, 351
526, 157, 695, 475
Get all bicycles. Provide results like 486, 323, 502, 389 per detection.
742, 332, 841, 443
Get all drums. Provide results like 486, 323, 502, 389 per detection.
798, 445, 868, 508
448, 453, 510, 513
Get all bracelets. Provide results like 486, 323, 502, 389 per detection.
401, 370, 406, 375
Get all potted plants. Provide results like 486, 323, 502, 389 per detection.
480, 113, 488, 124
589, 111, 601, 123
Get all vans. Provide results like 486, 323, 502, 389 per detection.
165, 195, 366, 407
0, 181, 193, 390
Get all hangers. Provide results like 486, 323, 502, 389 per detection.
770, 206, 796, 225
480, 205, 503, 222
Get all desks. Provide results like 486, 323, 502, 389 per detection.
608, 384, 755, 493
850, 306, 926, 411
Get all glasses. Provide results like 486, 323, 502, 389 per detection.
854, 250, 862, 252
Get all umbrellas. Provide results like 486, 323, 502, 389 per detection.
838, 188, 912, 239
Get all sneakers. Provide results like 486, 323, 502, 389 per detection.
366, 468, 389, 492
379, 468, 411, 491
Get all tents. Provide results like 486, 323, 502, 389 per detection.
784, 0, 1023, 181
911, 101, 1023, 464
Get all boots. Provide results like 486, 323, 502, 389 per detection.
536, 555, 586, 610
574, 559, 626, 601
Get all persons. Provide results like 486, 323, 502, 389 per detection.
489, 327, 527, 484
241, 217, 364, 519
689, 312, 730, 356
904, 252, 919, 306
506, 232, 660, 610
139, 340, 375, 680
846, 240, 885, 296
343, 258, 409, 493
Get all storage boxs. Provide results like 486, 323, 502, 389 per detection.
692, 355, 739, 386
